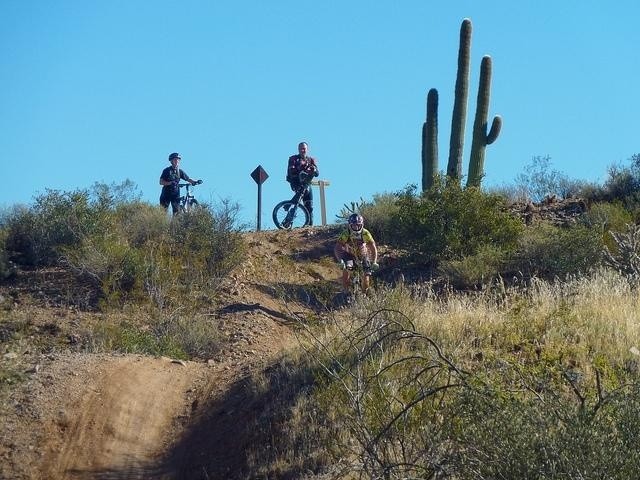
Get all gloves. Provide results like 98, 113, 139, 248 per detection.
198, 180, 203, 184
170, 181, 177, 185
372, 262, 379, 271
336, 259, 345, 271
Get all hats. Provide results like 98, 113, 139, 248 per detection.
169, 153, 181, 160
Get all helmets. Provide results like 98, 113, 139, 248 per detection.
348, 214, 364, 235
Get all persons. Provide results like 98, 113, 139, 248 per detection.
160, 153, 203, 216
287, 142, 320, 228
333, 213, 380, 298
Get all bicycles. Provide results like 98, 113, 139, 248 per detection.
174, 180, 201, 220
339, 264, 372, 304
274, 177, 310, 229
